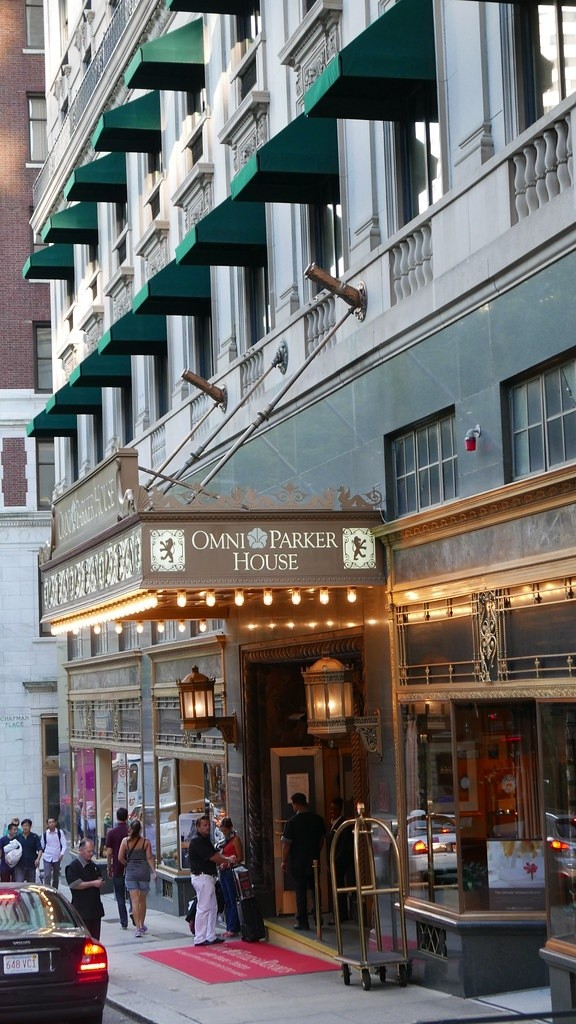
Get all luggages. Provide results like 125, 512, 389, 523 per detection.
231, 862, 267, 942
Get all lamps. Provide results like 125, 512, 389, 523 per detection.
175, 664, 237, 751
301, 648, 383, 762
465, 424, 481, 452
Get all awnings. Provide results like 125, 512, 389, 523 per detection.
124, 18, 205, 93
27, 409, 77, 437
175, 194, 268, 267
69, 349, 131, 388
64, 152, 127, 203
46, 382, 102, 415
167, 0, 254, 16
301, 0, 438, 123
230, 112, 340, 204
41, 202, 98, 245
132, 258, 211, 317
22, 244, 74, 280
91, 90, 162, 154
97, 309, 167, 355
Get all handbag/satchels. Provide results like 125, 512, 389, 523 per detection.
35, 866, 44, 885
233, 865, 255, 901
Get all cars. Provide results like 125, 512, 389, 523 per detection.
371, 810, 459, 891
0, 879, 110, 1024
545, 807, 576, 905
137, 783, 219, 827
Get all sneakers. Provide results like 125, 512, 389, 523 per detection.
140, 926, 148, 932
134, 930, 141, 937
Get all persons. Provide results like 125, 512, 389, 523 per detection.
105, 807, 136, 930
187, 815, 244, 946
280, 793, 325, 929
118, 820, 156, 938
0, 816, 67, 890
327, 796, 351, 925
65, 838, 105, 941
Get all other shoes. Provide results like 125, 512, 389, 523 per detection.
208, 937, 226, 944
195, 939, 213, 946
315, 921, 324, 926
129, 913, 137, 926
294, 923, 309, 930
223, 931, 239, 937
327, 920, 335, 926
120, 925, 128, 930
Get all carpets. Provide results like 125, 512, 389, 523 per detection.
134, 940, 344, 985
